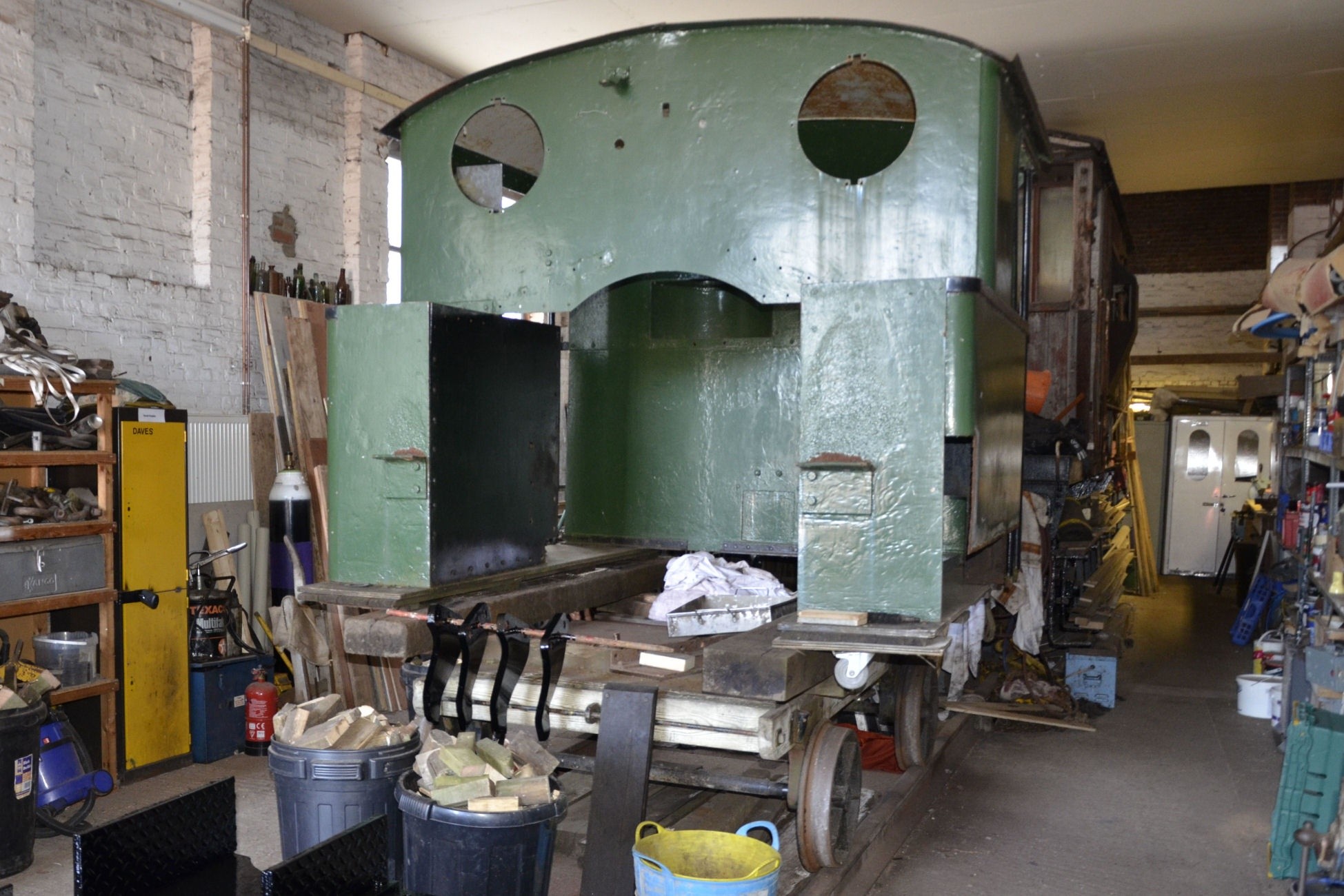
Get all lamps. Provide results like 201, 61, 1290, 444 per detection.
266, 446, 313, 549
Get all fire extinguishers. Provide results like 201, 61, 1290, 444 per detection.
245, 664, 280, 755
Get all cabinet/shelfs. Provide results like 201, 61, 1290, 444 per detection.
45, 404, 194, 780
1269, 321, 1339, 717
1297, 321, 1344, 714
1, 375, 128, 797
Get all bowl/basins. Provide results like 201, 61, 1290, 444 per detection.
33, 631, 98, 687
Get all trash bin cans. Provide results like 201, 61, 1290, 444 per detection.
270, 726, 424, 858
393, 770, 571, 895
0, 698, 48, 879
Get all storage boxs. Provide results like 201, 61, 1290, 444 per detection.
1060, 647, 1116, 712
186, 655, 262, 765
1264, 701, 1344, 878
1231, 572, 1271, 647
1279, 506, 1299, 546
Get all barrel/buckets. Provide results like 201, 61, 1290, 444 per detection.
1236, 630, 1284, 727
268, 722, 783, 896
